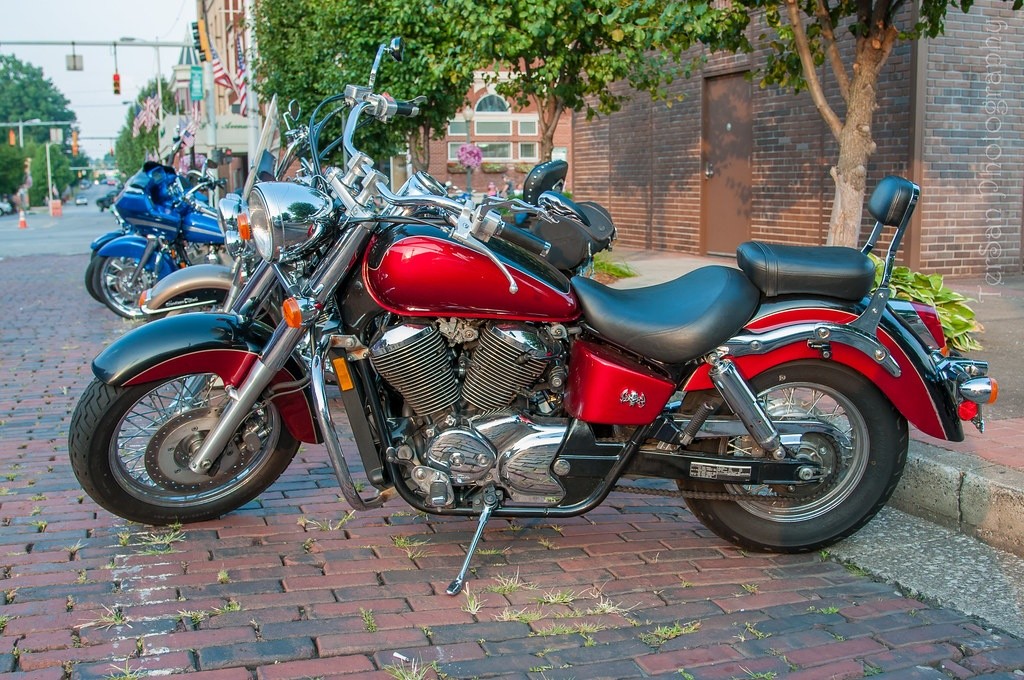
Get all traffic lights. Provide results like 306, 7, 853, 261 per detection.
113, 74, 121, 95
226, 149, 232, 163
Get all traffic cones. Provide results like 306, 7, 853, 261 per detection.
18, 209, 29, 229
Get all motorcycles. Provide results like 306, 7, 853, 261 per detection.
70, 38, 998, 594
84, 121, 570, 322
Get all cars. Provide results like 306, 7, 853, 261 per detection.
75, 195, 88, 206
100, 180, 107, 184
80, 181, 90, 189
107, 180, 116, 186
